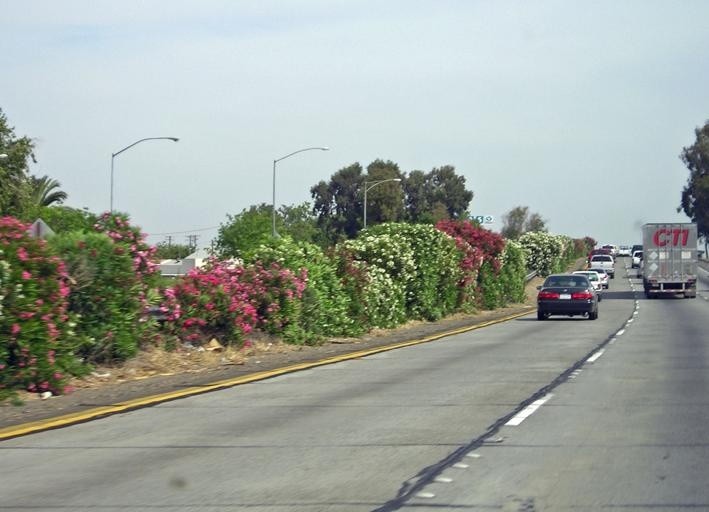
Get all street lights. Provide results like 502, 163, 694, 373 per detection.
363, 178, 401, 228
111, 137, 178, 213
273, 147, 329, 232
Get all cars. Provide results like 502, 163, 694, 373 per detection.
537, 244, 643, 320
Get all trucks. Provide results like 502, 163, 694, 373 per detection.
643, 223, 697, 298
